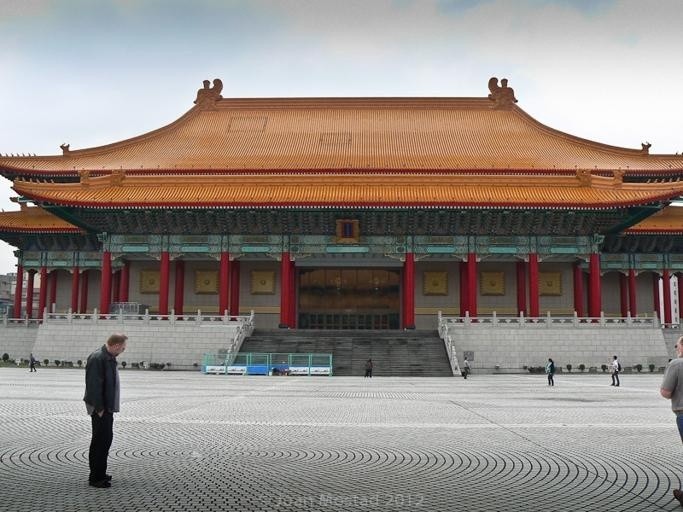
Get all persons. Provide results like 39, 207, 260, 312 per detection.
82, 334, 130, 490
463, 357, 471, 379
547, 358, 555, 386
363, 358, 372, 377
29, 352, 36, 372
659, 336, 683, 507
608, 354, 620, 387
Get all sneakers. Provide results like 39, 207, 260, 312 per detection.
672, 488, 683, 507
89, 474, 112, 488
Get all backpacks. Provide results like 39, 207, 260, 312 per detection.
618, 362, 621, 373
